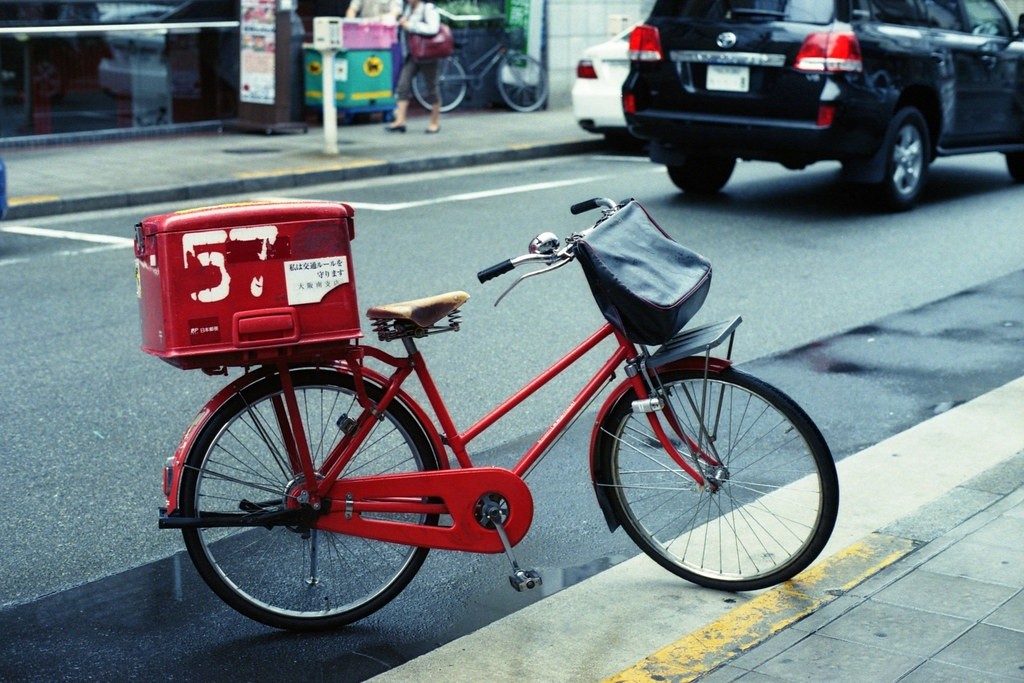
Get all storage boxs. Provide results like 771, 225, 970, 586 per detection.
132, 200, 365, 358
342, 22, 398, 49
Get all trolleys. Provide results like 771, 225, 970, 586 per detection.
302, 14, 405, 126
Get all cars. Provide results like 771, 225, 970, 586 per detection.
620, 1, 1024, 217
0, 0, 105, 103
98, 0, 239, 110
571, 18, 649, 154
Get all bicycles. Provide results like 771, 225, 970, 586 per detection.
154, 197, 842, 632
411, 21, 549, 113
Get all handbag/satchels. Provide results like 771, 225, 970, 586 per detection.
573, 197, 713, 347
408, 3, 454, 64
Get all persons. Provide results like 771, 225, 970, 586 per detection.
345, 0, 403, 22
385, 0, 441, 134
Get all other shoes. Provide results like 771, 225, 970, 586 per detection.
385, 125, 406, 132
426, 126, 440, 133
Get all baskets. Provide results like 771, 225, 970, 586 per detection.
495, 25, 525, 50
